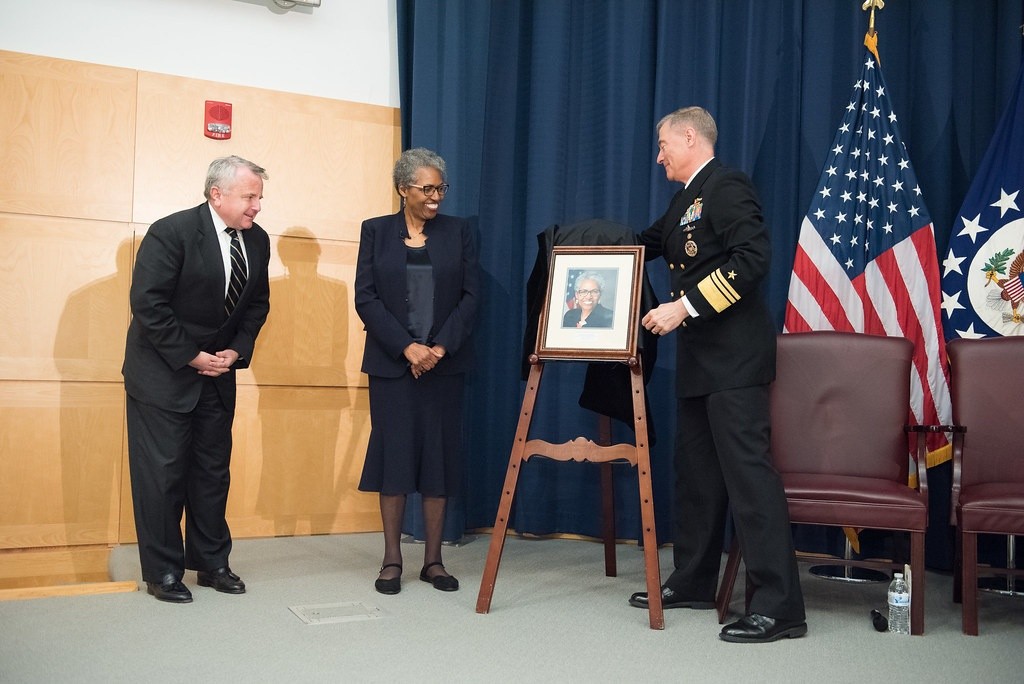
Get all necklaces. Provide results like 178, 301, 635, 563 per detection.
410, 230, 422, 237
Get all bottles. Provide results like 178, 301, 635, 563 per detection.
887, 573, 910, 634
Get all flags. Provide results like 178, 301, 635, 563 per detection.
566, 270, 584, 310
938, 66, 1024, 344
780, 33, 954, 554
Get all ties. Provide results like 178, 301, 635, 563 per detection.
223, 228, 248, 323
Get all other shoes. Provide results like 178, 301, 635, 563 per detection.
419, 563, 460, 591
374, 563, 403, 594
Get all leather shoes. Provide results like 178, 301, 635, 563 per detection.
196, 567, 245, 594
720, 610, 808, 645
627, 584, 720, 610
147, 574, 194, 603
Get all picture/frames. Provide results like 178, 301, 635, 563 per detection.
532, 245, 645, 361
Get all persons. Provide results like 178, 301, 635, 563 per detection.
354, 148, 483, 597
118, 153, 274, 604
563, 272, 613, 328
627, 105, 808, 643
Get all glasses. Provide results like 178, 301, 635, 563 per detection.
576, 289, 601, 297
409, 182, 449, 196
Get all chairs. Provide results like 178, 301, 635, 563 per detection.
718, 330, 926, 635
949, 335, 1023, 634
